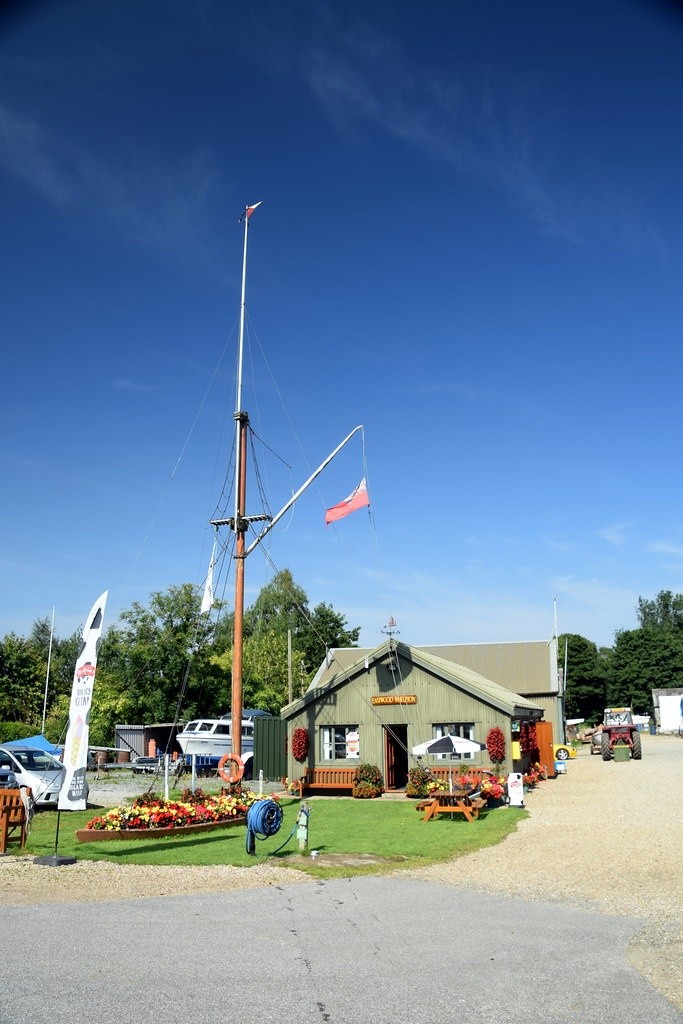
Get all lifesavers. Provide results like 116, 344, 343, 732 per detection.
218, 754, 244, 782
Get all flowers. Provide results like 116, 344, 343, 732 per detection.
405, 767, 447, 799
456, 772, 508, 800
352, 764, 385, 799
288, 779, 300, 793
523, 762, 547, 786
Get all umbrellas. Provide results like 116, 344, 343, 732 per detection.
412, 732, 486, 795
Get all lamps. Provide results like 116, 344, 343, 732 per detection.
387, 663, 399, 672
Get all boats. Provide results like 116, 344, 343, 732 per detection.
176, 710, 272, 767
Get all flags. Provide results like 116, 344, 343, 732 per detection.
324, 475, 370, 528
57, 590, 109, 810
200, 541, 216, 614
247, 201, 262, 220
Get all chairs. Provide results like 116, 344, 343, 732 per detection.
0, 786, 32, 855
11, 754, 23, 771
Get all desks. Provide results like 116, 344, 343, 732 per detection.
422, 789, 480, 822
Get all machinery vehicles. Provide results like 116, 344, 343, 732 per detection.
601, 708, 642, 761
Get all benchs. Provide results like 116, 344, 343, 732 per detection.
471, 799, 487, 808
430, 768, 491, 782
416, 800, 432, 808
298, 768, 360, 797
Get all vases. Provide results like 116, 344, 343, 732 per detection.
523, 783, 530, 792
488, 797, 501, 807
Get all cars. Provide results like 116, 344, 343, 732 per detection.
0, 744, 89, 806
553, 743, 577, 760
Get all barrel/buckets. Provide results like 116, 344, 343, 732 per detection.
614, 745, 629, 762
96, 751, 108, 763
116, 751, 129, 763
